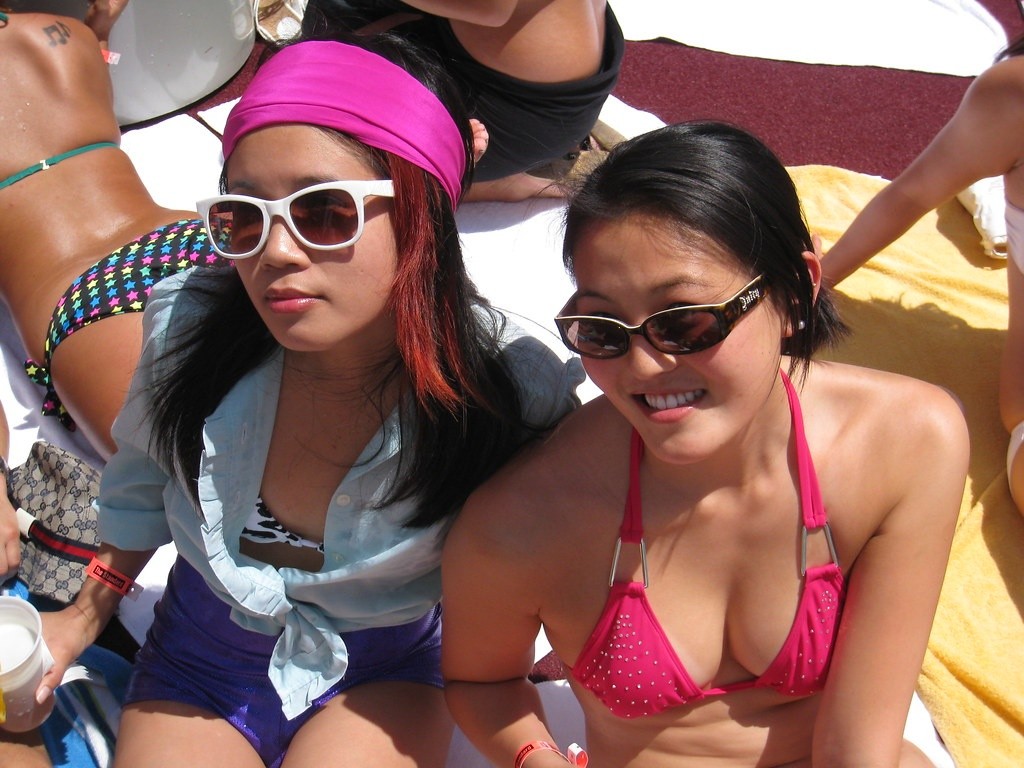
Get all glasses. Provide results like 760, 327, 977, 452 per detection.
554, 269, 775, 359
196, 180, 396, 259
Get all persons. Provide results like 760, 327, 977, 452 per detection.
0, 0, 1024, 768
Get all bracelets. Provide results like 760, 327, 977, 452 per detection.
84, 557, 144, 603
513, 740, 588, 768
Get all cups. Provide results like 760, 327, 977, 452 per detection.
0, 595, 55, 733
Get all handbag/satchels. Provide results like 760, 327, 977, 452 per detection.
14, 442, 102, 606
